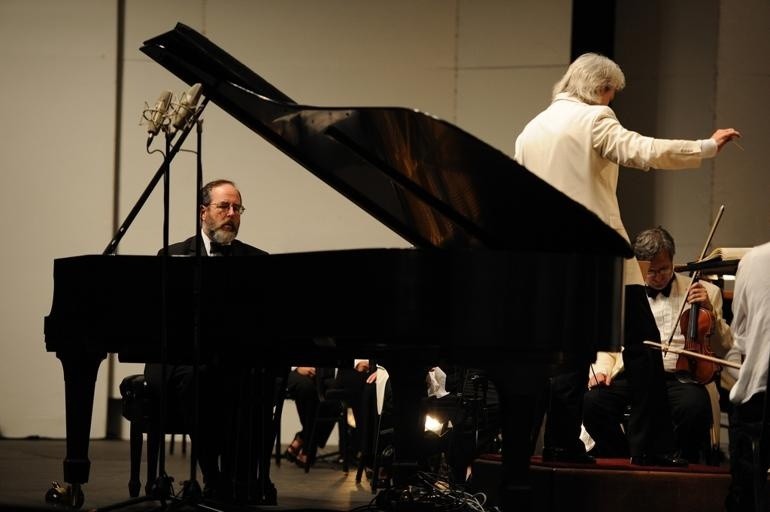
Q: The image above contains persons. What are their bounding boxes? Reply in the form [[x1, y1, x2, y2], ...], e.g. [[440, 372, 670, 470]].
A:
[[514, 49, 741, 472], [582, 223, 735, 464], [282, 355, 382, 472], [724, 242, 770, 510], [142, 177, 271, 498]]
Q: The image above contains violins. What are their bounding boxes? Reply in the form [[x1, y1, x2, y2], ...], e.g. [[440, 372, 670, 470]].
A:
[[674, 255, 722, 383]]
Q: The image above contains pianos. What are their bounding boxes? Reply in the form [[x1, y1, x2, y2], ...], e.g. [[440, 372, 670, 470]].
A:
[[45, 14, 636, 509]]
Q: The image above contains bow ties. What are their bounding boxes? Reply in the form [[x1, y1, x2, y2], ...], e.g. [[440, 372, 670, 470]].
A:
[[646, 281, 673, 300], [208, 241, 233, 256]]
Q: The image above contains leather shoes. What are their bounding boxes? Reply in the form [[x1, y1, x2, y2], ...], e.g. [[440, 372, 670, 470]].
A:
[[543, 448, 596, 465], [628, 450, 689, 466]]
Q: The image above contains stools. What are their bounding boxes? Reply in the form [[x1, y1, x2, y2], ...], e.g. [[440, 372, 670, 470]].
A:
[[120, 372, 248, 498]]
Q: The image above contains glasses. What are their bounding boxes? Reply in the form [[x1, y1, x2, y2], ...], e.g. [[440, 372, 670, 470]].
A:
[[207, 202, 245, 215]]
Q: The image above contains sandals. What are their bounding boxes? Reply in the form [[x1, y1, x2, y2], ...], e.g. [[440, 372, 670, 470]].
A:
[[295, 444, 309, 469], [284, 432, 304, 462]]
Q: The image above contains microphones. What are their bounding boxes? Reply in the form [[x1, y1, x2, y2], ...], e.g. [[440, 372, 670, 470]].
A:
[[147, 91, 172, 133], [172, 83, 205, 129]]
